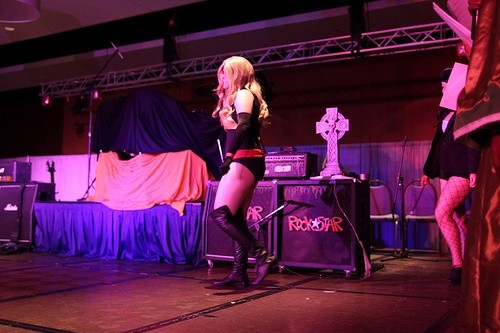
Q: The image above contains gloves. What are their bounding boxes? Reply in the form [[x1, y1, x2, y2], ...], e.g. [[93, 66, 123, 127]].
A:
[[218, 157, 233, 175]]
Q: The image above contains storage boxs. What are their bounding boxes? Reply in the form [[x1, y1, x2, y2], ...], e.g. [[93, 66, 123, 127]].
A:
[[263, 153, 318, 179], [0, 160, 32, 184]]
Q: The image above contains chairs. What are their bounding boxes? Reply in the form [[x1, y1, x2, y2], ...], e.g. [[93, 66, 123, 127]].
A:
[[369, 179, 471, 258]]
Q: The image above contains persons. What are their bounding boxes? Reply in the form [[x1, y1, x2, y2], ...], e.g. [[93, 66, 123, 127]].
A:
[[211, 56, 277, 290], [421, 69, 481, 285]]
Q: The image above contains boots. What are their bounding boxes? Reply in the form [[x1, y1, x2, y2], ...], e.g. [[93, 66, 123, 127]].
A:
[[213, 208, 249, 287], [208, 205, 278, 285]]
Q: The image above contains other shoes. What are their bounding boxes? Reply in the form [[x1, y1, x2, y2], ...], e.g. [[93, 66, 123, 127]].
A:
[[447, 268, 462, 285]]
